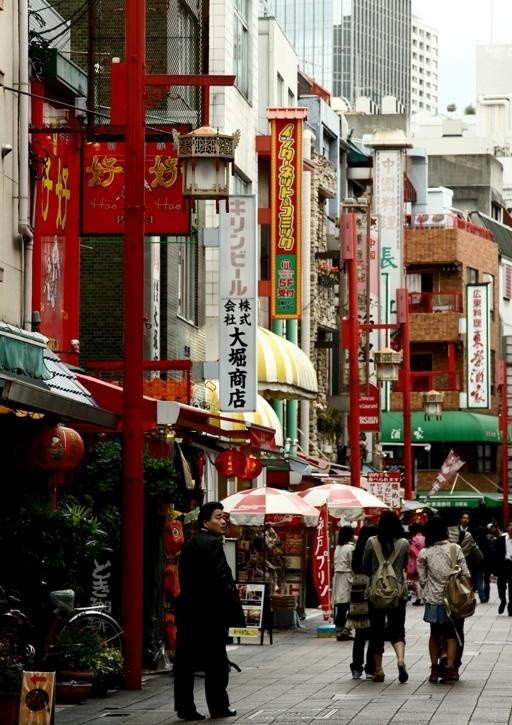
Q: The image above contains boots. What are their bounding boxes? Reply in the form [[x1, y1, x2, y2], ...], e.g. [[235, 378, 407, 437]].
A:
[[428, 665, 454, 684]]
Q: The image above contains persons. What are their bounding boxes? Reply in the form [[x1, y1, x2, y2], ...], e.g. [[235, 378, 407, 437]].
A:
[[332, 511, 512, 683], [174, 500, 246, 720]]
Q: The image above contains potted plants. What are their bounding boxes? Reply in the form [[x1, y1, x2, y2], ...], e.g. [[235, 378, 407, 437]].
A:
[[0, 643, 40, 725], [58, 628, 108, 697]]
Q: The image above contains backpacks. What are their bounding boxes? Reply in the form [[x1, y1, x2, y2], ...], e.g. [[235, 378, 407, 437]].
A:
[[444, 544, 476, 620], [368, 536, 403, 607]]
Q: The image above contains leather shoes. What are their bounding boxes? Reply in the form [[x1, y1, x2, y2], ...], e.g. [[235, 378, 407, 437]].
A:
[[211, 707, 236, 718], [498, 601, 506, 614], [183, 710, 205, 721]]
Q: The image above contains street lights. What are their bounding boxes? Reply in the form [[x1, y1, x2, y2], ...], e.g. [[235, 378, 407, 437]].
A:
[[119, 69, 243, 698], [395, 368, 451, 502], [346, 319, 408, 490]]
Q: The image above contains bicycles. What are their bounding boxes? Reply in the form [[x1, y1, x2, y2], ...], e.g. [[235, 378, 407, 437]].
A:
[[1, 584, 123, 671]]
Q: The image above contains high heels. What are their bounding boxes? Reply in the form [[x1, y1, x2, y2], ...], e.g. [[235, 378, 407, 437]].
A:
[[372, 670, 385, 682], [398, 661, 409, 683]]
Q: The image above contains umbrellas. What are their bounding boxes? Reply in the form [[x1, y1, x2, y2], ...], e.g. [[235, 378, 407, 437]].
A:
[[217, 483, 320, 583], [297, 481, 392, 521]]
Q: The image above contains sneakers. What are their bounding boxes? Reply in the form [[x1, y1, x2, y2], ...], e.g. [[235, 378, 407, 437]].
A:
[[366, 672, 372, 680], [336, 631, 343, 641], [342, 627, 355, 641], [351, 669, 362, 680]]
